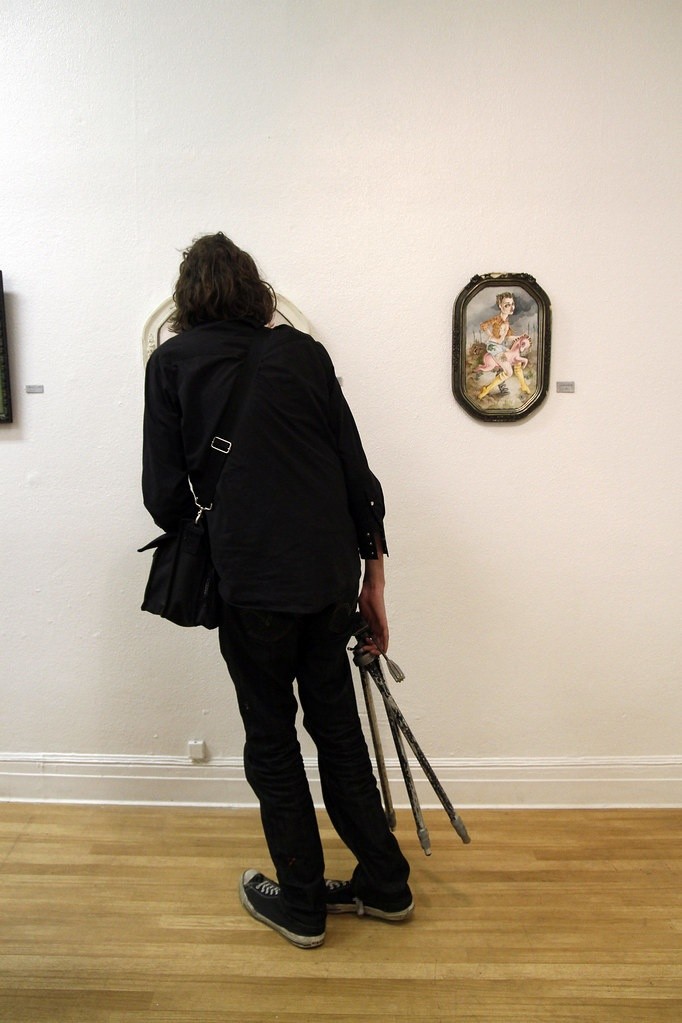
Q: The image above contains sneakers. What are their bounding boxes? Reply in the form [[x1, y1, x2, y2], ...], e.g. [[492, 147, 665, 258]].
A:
[[240, 867, 326, 947], [322, 876, 414, 921]]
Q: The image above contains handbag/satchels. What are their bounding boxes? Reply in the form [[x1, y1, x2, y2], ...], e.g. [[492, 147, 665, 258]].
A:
[[136, 526, 218, 630]]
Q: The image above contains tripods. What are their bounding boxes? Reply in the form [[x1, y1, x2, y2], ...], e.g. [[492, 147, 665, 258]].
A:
[[349, 611, 472, 857]]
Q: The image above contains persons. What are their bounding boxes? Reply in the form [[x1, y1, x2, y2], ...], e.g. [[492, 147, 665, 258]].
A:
[[141, 232, 416, 948]]
